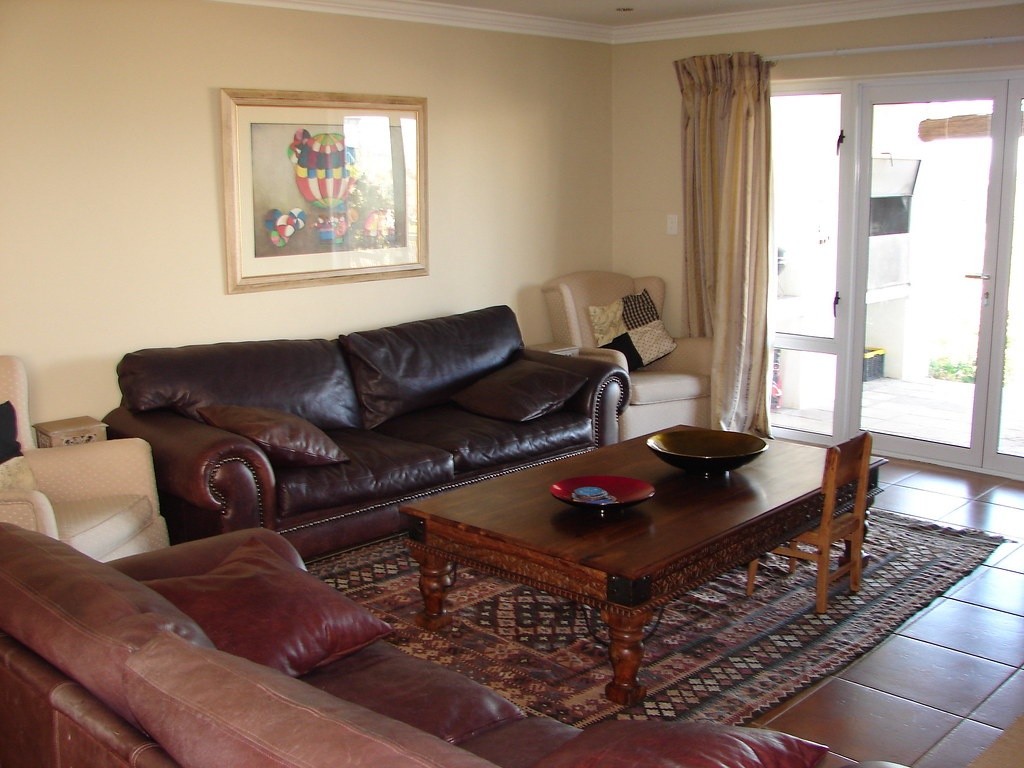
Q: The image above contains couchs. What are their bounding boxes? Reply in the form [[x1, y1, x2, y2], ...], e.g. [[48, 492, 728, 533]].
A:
[[1, 354, 169, 563], [0, 522, 916, 768], [542, 270, 713, 440], [101, 305, 631, 563]]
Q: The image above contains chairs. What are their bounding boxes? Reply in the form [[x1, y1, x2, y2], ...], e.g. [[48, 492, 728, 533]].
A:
[[747, 431, 873, 612]]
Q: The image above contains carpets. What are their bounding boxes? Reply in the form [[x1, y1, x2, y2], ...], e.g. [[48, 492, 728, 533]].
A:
[[308, 506, 1016, 731]]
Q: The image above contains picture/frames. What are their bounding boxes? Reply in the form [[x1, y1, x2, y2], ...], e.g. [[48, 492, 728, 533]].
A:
[[220, 89, 430, 295]]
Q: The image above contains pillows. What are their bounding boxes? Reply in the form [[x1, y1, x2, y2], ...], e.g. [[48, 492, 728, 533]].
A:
[[538, 722, 830, 768], [100, 526, 394, 677], [196, 406, 350, 465], [451, 358, 587, 420], [588, 288, 677, 374]]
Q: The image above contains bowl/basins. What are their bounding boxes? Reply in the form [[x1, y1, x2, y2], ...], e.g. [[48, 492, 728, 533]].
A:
[[646, 428, 769, 479], [548, 475, 655, 512]]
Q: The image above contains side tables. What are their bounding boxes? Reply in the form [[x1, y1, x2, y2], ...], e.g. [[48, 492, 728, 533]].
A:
[[526, 342, 577, 357]]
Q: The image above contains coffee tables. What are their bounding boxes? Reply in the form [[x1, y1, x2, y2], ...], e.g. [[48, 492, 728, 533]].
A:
[[398, 424, 890, 708]]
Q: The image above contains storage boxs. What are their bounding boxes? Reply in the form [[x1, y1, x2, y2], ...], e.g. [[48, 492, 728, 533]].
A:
[[864, 348, 884, 381]]
